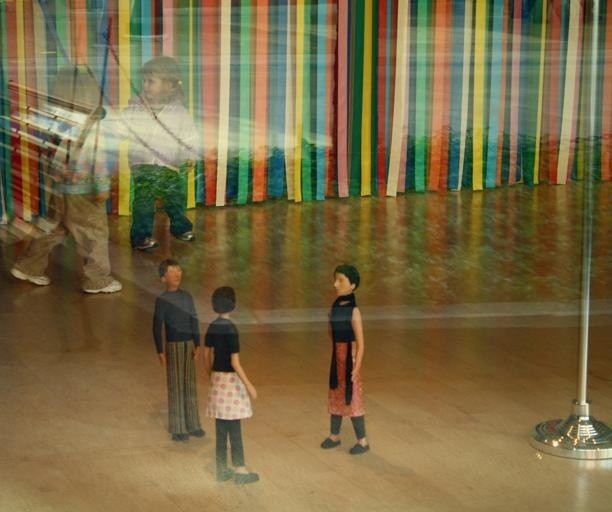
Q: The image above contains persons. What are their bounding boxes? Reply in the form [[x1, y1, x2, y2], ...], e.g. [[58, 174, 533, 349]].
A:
[[153, 260, 205, 442], [204, 286, 263, 485], [321, 263, 371, 454], [113, 59, 199, 248], [10, 63, 122, 294]]
